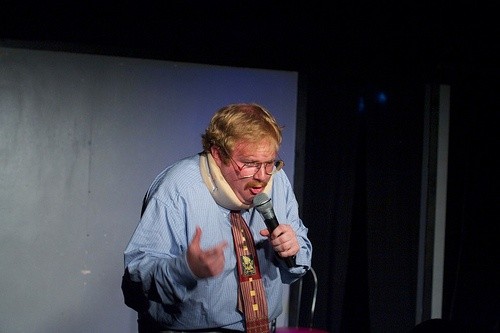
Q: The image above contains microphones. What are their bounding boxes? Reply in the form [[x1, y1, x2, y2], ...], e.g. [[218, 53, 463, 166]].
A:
[[254, 193, 295, 269]]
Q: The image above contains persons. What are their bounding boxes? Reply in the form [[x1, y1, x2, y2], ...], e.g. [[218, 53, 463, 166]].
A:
[[123, 103, 313, 333]]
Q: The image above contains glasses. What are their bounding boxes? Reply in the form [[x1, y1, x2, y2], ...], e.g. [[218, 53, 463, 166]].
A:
[[222, 142, 285, 177]]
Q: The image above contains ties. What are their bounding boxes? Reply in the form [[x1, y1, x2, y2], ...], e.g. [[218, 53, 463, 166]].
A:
[[231, 210, 269, 333]]
